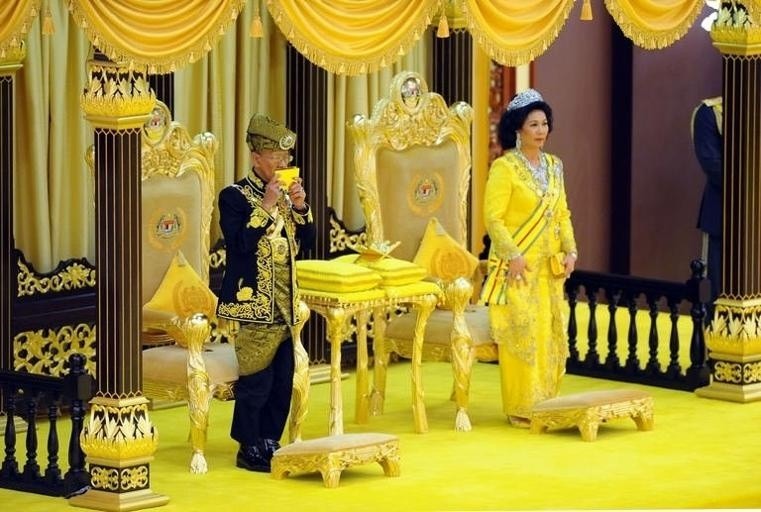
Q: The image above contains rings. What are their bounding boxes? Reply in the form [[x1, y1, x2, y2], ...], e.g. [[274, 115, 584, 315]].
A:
[[516, 273, 522, 280], [301, 186, 304, 193]]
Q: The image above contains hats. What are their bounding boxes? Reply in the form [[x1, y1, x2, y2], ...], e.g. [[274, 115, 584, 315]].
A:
[[246, 113, 297, 153]]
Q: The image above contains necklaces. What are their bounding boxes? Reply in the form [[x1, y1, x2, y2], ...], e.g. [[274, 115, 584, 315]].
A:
[[520, 150, 550, 192]]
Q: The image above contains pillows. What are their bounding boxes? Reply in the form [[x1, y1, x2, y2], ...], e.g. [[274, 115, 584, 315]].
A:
[[411, 217, 481, 310], [139, 249, 219, 348]]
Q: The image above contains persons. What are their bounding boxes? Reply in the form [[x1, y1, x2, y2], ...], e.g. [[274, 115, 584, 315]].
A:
[[692, 93, 723, 372], [480, 90, 578, 430], [214, 112, 313, 472]]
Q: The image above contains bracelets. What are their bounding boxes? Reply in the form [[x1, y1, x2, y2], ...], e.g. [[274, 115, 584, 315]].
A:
[[571, 252, 578, 261], [509, 253, 521, 261]]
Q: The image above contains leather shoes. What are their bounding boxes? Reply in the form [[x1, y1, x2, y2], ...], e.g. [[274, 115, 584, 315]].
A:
[[237, 437, 281, 472], [507, 415, 530, 429]]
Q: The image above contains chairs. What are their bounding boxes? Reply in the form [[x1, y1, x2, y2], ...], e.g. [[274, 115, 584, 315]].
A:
[[343, 71, 573, 433], [84, 100, 312, 476]]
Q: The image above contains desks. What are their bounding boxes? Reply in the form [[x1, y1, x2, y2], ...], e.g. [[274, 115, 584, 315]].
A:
[[293, 281, 438, 436]]
